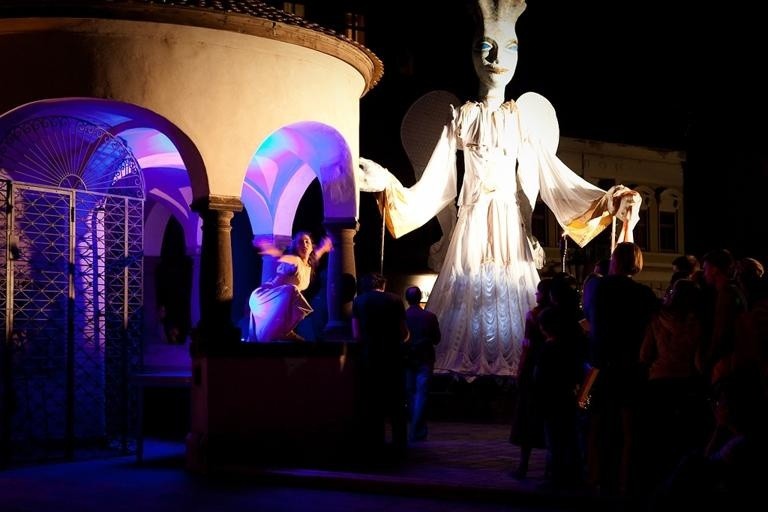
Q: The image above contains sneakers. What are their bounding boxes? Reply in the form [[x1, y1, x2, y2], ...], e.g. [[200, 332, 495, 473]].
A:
[[512, 463, 529, 479], [409, 426, 429, 441]]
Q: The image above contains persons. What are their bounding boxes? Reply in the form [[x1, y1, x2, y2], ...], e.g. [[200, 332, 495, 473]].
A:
[[354, 1, 643, 388], [252, 229, 332, 318], [248, 261, 314, 344], [496, 237, 767, 511], [400, 286, 441, 442], [351, 271, 406, 343]]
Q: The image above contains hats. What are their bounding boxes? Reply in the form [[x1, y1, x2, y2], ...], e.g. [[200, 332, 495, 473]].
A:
[[538, 271, 581, 299], [702, 248, 737, 281]]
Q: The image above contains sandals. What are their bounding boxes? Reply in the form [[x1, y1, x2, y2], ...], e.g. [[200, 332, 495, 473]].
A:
[[280, 329, 305, 342]]
